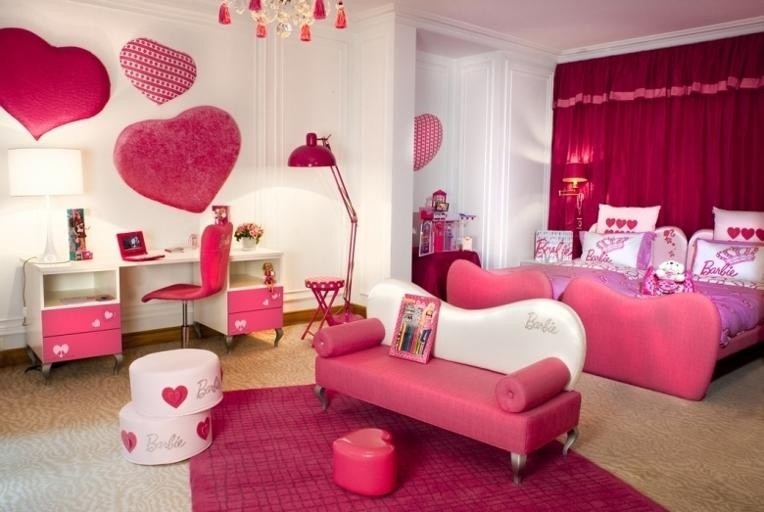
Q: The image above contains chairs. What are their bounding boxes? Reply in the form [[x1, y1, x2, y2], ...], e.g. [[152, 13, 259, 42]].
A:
[[142, 223, 233, 348]]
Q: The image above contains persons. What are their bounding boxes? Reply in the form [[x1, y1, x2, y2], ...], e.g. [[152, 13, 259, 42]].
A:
[[263, 263, 275, 293], [74, 212, 87, 250], [421, 303, 437, 355]]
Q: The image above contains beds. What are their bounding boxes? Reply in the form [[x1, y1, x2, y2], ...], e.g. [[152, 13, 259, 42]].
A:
[[447, 224, 764, 401]]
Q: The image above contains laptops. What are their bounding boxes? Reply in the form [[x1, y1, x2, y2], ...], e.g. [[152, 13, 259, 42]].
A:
[[115, 231, 167, 263]]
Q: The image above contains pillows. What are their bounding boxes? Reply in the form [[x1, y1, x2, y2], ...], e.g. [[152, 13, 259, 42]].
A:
[[578, 231, 656, 271], [691, 238, 764, 282], [596, 203, 662, 233], [712, 205, 764, 243]]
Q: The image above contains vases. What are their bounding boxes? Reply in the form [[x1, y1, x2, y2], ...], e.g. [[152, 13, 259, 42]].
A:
[[240, 238, 256, 250]]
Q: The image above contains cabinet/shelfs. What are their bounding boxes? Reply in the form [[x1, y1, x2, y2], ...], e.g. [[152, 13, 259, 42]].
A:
[[23, 248, 284, 381]]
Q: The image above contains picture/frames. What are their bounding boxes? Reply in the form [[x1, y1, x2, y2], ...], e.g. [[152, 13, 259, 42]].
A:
[[389, 294, 440, 365]]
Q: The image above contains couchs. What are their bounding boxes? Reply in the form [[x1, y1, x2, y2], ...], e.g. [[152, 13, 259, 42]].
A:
[[314, 279, 587, 485]]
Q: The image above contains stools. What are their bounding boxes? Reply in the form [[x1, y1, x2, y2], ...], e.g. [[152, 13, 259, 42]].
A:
[[301, 278, 345, 347], [120, 405, 212, 466], [333, 428, 398, 497], [129, 349, 224, 417]]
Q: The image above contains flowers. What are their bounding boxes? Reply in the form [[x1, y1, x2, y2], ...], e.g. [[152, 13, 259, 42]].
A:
[[235, 223, 263, 244]]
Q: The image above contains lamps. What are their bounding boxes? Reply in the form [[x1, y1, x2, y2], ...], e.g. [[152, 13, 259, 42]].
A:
[[218, 0, 347, 41], [8, 148, 85, 265], [288, 133, 364, 323], [558, 162, 590, 230]]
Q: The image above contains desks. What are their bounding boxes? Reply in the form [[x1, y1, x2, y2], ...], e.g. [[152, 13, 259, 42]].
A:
[[413, 246, 481, 301]]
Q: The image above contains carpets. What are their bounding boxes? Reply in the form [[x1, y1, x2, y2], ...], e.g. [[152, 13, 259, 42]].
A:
[[189, 384, 671, 512]]
